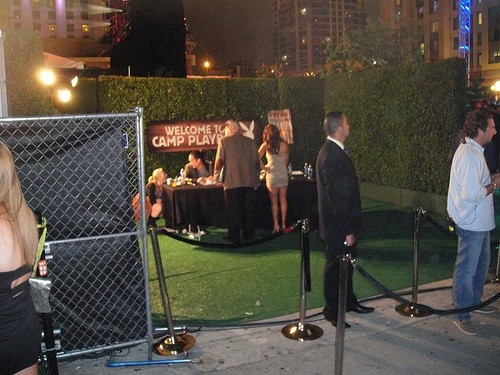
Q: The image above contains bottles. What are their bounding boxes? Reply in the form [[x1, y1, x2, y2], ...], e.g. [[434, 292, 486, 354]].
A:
[[304, 163, 308, 177], [180, 168, 186, 184], [308, 165, 313, 180], [288, 163, 292, 177]]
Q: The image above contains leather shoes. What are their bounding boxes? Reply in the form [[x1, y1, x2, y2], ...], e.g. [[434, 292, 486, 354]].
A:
[[346, 304, 375, 314], [324, 311, 351, 329]]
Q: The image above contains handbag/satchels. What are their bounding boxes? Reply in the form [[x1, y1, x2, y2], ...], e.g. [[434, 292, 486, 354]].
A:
[[132, 181, 154, 223], [218, 164, 225, 182], [29, 277, 52, 313]]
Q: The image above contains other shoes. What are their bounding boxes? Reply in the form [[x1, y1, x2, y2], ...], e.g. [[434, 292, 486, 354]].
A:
[[246, 239, 252, 242], [222, 236, 238, 244], [475, 306, 495, 313], [454, 319, 478, 336]]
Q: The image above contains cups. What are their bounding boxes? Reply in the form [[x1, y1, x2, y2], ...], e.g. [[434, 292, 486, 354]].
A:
[[166, 177, 192, 187]]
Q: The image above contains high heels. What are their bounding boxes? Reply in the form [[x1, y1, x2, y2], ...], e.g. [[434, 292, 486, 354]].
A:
[[280, 225, 285, 231], [272, 226, 280, 234]]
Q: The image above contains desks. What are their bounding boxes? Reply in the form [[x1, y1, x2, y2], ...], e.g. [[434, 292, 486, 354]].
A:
[[163, 173, 316, 232]]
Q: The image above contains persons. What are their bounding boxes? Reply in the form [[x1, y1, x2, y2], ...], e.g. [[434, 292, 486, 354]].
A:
[[146, 168, 169, 229], [182, 151, 210, 185], [447, 109, 500, 334], [314, 112, 374, 328], [0, 140, 41, 375], [258, 123, 289, 235], [212, 120, 260, 242]]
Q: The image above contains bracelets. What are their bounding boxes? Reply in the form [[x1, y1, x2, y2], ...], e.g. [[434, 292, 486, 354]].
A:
[[492, 183, 496, 190]]
